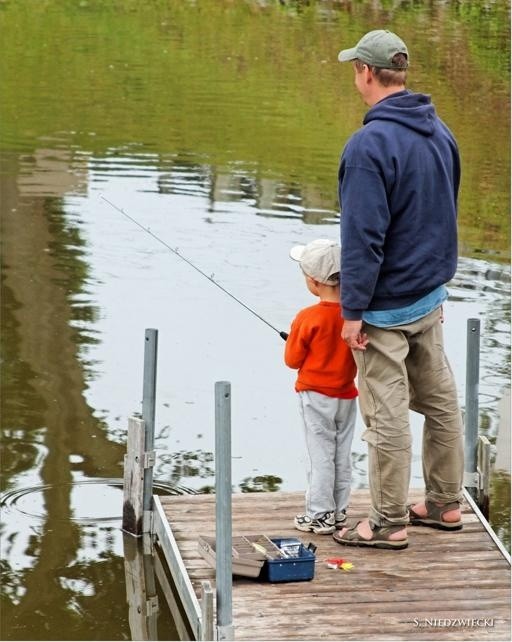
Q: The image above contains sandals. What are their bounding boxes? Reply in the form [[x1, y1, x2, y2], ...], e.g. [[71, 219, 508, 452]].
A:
[[407, 498, 463, 531], [333, 521, 408, 551]]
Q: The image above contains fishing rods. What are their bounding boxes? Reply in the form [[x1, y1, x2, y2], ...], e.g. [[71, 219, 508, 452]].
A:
[[100, 195, 289, 342]]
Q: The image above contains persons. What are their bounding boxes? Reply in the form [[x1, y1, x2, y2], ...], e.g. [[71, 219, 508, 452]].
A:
[[284, 237, 358, 535], [336, 30, 464, 548]]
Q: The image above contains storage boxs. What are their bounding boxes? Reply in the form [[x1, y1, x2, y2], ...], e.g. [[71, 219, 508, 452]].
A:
[[197, 532, 319, 583]]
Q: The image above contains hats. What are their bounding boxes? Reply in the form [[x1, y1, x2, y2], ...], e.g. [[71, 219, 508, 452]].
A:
[[290, 239, 342, 286], [338, 29, 409, 69]]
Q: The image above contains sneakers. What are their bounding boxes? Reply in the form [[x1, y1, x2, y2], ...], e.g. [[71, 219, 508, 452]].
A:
[[334, 510, 348, 529], [294, 512, 336, 535]]
